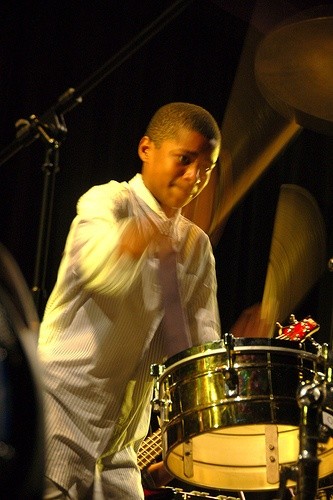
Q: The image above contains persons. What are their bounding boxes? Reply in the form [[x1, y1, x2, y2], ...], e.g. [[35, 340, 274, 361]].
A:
[[36, 101, 223, 500]]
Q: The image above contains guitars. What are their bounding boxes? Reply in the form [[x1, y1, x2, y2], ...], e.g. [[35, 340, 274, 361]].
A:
[[138, 315, 318, 469]]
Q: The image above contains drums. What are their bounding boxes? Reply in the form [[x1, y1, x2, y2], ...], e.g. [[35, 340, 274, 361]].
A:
[[151, 332, 331, 491]]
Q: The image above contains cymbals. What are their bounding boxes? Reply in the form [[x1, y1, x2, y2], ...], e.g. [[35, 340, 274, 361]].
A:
[[255, 17, 333, 121]]
